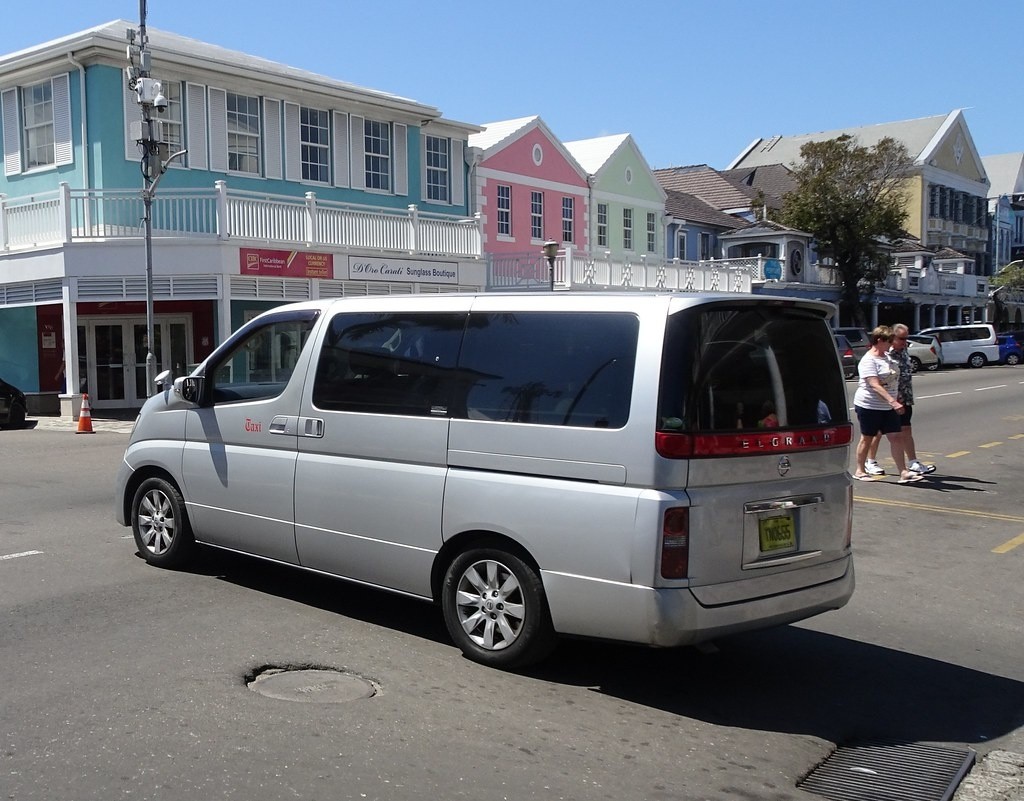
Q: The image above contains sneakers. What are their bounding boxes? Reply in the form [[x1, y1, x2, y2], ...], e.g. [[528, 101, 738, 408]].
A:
[[908, 461, 936, 473], [864, 460, 884, 475]]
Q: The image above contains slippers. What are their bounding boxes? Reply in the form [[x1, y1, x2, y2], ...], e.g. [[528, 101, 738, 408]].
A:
[[851, 472, 877, 482], [896, 470, 924, 484]]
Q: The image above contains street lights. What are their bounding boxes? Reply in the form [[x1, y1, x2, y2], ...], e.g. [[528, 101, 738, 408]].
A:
[[542, 238, 559, 292]]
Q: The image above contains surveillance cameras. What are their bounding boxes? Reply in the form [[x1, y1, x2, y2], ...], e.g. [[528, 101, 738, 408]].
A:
[[153, 95, 168, 113]]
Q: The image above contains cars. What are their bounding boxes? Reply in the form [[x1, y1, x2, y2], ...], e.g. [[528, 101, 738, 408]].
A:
[[906, 341, 938, 375], [995, 334, 1024, 365], [833, 327, 871, 362], [0, 379, 27, 430], [833, 334, 856, 380], [908, 335, 945, 371]]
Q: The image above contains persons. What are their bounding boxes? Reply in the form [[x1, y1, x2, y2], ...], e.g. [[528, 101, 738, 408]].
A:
[[851, 323, 936, 484]]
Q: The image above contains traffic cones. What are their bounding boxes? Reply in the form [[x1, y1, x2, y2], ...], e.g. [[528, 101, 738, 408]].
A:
[[75, 393, 96, 433]]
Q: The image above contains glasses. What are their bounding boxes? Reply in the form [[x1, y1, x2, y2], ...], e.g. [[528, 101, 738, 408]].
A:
[[882, 339, 893, 344], [895, 336, 907, 340]]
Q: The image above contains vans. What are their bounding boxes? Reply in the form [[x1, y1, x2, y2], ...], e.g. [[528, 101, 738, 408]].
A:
[[116, 290, 857, 667], [916, 324, 1001, 369]]
